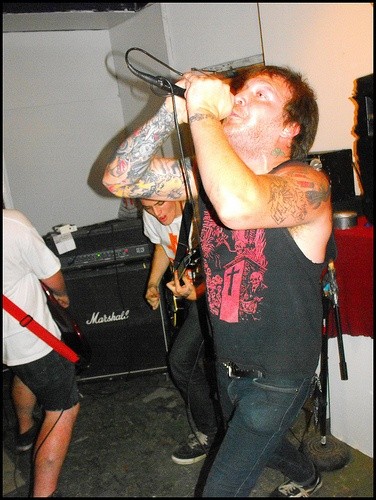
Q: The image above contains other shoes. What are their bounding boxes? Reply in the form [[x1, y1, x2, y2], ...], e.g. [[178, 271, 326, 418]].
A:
[[15, 417, 45, 451]]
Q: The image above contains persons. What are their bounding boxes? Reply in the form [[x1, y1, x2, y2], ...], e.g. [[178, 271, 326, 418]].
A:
[[138, 197, 225, 465], [2, 209, 80, 498], [101, 65, 332, 498]]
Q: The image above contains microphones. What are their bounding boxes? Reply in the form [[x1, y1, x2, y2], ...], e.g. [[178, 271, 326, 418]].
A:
[[157, 77, 228, 125]]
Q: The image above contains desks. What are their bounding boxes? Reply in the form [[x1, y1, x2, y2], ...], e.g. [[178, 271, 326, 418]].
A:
[[328, 217, 373, 459]]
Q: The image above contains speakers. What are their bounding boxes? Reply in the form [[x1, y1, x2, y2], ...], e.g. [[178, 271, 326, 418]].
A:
[[43, 258, 170, 384]]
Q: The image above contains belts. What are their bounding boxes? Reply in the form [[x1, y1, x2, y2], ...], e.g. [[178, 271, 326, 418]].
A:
[[219, 359, 262, 380]]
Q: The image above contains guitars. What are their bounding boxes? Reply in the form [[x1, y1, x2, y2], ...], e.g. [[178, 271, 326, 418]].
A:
[[158, 245, 203, 326], [40, 281, 89, 354]]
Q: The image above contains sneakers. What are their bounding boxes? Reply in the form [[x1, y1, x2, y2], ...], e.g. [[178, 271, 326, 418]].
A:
[[172, 431, 212, 466], [269, 469, 323, 498]]
